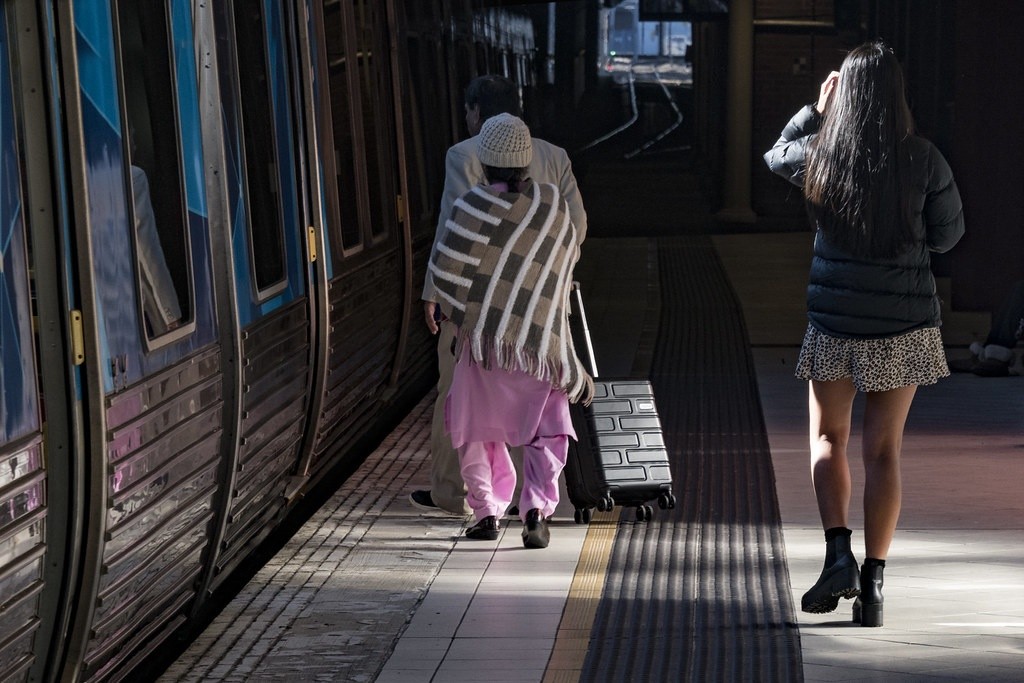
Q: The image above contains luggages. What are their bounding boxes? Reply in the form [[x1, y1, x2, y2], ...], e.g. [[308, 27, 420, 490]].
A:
[[563, 269, 677, 524]]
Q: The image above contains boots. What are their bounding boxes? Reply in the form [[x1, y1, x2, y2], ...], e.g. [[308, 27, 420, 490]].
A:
[[800, 529, 885, 628]]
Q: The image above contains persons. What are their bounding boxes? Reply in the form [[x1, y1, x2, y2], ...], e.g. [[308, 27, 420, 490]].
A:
[[433, 109, 593, 548], [942, 284, 1023, 376], [763, 33, 965, 626], [410, 75, 587, 517]]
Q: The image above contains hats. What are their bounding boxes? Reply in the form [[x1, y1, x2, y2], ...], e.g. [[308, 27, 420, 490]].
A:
[[475, 112, 533, 167]]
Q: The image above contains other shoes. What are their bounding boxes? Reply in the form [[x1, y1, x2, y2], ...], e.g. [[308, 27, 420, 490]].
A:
[[408, 489, 462, 517], [521, 507, 549, 547], [466, 515, 499, 541]]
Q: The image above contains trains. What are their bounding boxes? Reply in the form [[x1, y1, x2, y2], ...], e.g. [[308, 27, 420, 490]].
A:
[[0, 0, 588, 683]]
